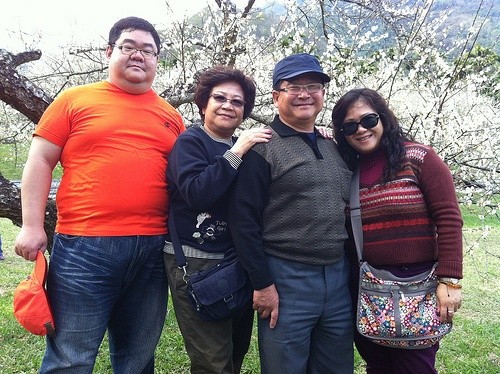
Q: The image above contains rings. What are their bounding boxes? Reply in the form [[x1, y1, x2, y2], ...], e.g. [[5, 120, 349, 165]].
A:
[[447, 311, 454, 313]]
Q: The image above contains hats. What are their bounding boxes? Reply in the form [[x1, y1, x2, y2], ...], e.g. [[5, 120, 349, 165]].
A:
[[14, 250, 56, 337], [273, 53, 330, 89]]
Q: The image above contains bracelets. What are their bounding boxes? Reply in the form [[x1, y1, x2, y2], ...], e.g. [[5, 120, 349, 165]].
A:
[[440, 281, 462, 296], [440, 277, 459, 284]]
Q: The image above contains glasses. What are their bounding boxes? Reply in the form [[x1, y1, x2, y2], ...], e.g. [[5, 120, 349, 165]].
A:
[[208, 94, 247, 107], [340, 113, 379, 135], [276, 83, 325, 94], [113, 44, 157, 59]]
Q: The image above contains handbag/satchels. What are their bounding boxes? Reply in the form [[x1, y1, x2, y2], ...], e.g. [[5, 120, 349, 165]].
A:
[[356, 262, 452, 349], [187, 252, 253, 322]]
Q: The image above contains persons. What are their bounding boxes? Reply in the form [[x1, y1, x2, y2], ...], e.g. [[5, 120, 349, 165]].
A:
[[332, 89, 463, 374], [162, 65, 273, 374], [15, 17, 186, 374], [230, 54, 356, 374]]
